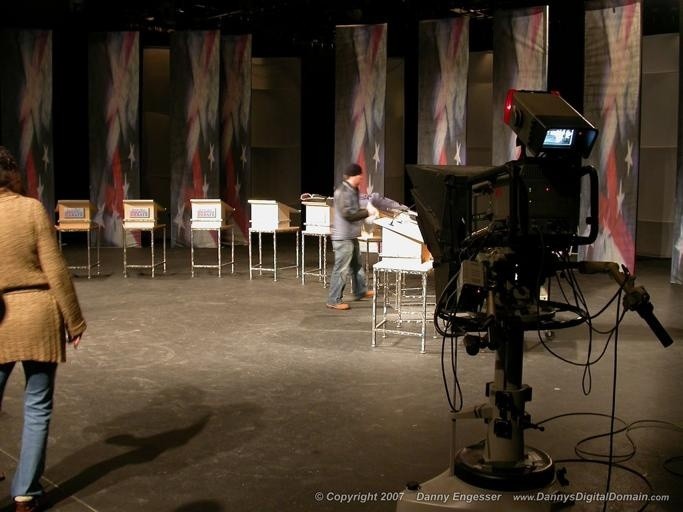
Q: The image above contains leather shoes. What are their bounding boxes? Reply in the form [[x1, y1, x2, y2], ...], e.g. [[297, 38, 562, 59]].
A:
[[362, 289, 374, 298], [326, 301, 350, 310]]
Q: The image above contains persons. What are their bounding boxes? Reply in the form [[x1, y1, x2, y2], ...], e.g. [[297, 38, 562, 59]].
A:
[[324, 163, 378, 310], [0, 148, 87, 511]]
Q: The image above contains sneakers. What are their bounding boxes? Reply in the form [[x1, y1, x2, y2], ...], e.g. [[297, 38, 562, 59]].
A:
[[14, 497, 38, 512]]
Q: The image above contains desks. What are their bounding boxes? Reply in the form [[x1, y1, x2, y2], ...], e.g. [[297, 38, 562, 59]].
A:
[[369, 253, 440, 355], [57, 224, 383, 295]]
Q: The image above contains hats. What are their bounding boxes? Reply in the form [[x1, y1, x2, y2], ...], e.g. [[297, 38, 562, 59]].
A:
[[342, 164, 362, 176]]
